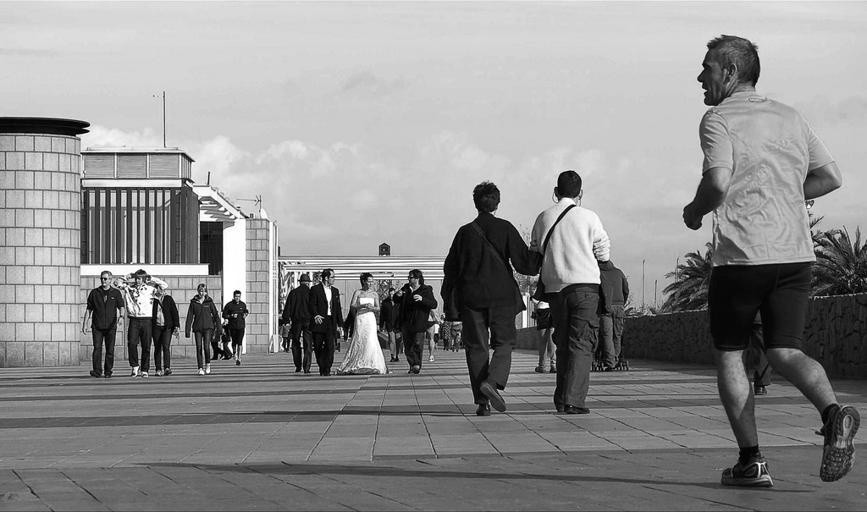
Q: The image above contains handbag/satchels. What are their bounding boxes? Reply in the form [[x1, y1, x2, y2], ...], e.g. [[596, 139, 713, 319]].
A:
[[534, 204, 577, 302], [535, 308, 556, 330]]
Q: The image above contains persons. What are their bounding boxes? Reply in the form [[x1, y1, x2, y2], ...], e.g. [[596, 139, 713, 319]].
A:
[[392, 269, 437, 375], [115, 270, 167, 377], [81, 270, 123, 379], [308, 267, 343, 378], [598, 259, 630, 367], [152, 285, 180, 377], [282, 276, 313, 375], [530, 269, 558, 373], [224, 290, 248, 366], [446, 182, 544, 416], [531, 171, 609, 415], [427, 308, 440, 363], [379, 287, 403, 363], [336, 272, 388, 375], [281, 321, 291, 352], [221, 319, 233, 360], [211, 310, 224, 363], [682, 35, 860, 487], [185, 283, 219, 375], [450, 321, 462, 353], [438, 313, 450, 351], [742, 311, 772, 394]]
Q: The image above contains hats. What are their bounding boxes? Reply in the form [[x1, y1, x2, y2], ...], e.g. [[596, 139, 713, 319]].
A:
[[298, 274, 312, 282]]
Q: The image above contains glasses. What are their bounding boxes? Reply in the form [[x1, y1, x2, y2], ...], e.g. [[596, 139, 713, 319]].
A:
[[100, 277, 108, 280], [330, 275, 335, 278], [409, 276, 415, 279]]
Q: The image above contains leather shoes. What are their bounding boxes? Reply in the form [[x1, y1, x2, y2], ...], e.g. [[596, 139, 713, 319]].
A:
[[755, 386, 768, 396]]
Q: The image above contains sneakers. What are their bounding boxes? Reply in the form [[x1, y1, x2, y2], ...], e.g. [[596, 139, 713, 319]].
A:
[[564, 405, 590, 414], [105, 372, 111, 378], [813, 405, 860, 482], [90, 371, 101, 377], [481, 380, 507, 412], [198, 370, 204, 375], [141, 372, 148, 378], [164, 368, 172, 375], [206, 365, 210, 374], [476, 406, 491, 416], [155, 369, 162, 376], [721, 456, 773, 488], [131, 366, 140, 378]]
